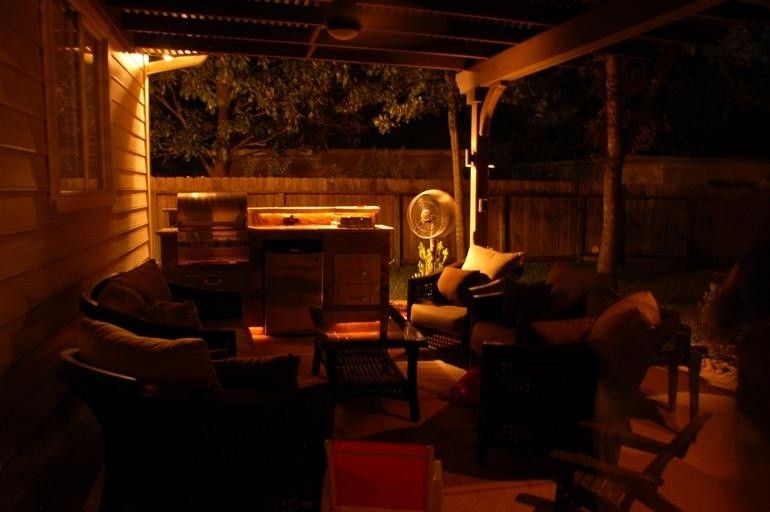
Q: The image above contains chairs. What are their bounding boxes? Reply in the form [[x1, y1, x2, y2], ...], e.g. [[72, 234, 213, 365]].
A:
[[80, 259, 246, 357], [649, 264, 741, 414], [462, 260, 613, 365], [408, 243, 527, 369], [476, 292, 662, 455], [547, 409, 712, 511], [56, 316, 334, 511]]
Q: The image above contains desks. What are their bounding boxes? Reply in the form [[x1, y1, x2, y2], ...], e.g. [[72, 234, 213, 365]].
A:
[[308, 305, 429, 419]]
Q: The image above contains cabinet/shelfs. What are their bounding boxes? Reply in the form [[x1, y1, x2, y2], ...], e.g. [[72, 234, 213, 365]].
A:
[[156, 192, 396, 335]]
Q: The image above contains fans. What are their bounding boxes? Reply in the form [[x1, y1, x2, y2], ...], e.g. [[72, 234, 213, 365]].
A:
[[407, 187, 458, 275]]
